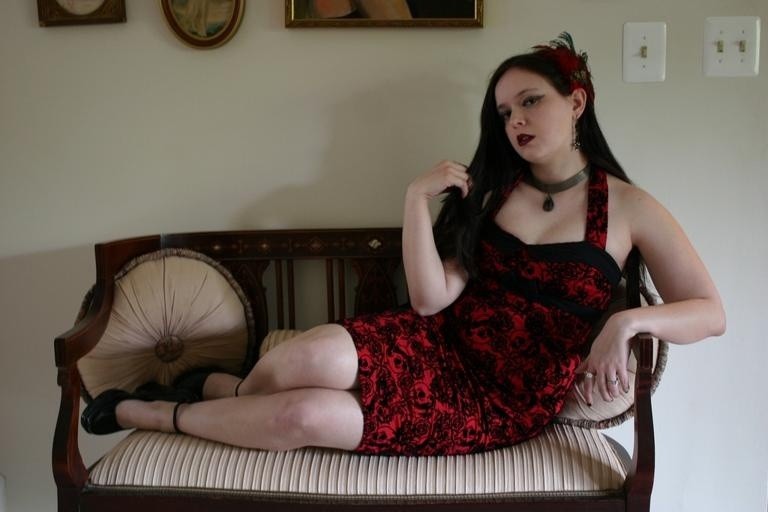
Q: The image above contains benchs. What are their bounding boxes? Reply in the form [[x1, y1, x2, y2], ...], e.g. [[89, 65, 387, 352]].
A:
[[51, 228, 654, 511]]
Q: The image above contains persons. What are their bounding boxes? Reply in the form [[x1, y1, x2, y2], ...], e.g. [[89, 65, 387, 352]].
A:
[[81, 32, 726, 455]]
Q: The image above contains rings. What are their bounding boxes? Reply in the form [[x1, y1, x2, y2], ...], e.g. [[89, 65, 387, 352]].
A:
[[607, 380, 618, 386], [582, 370, 594, 378]]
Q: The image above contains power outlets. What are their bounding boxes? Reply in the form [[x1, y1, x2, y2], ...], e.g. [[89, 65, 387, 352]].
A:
[[623, 17, 760, 84]]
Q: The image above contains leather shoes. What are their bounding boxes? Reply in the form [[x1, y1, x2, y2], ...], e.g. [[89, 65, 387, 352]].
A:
[[80, 381, 199, 434], [172, 366, 244, 401]]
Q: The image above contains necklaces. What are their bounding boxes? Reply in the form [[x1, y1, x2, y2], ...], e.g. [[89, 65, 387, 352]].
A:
[[521, 162, 591, 212]]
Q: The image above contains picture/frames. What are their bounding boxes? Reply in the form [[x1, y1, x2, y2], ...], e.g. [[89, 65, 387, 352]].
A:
[[37, 0, 128, 27], [285, 0, 485, 28], [160, 0, 247, 51]]
